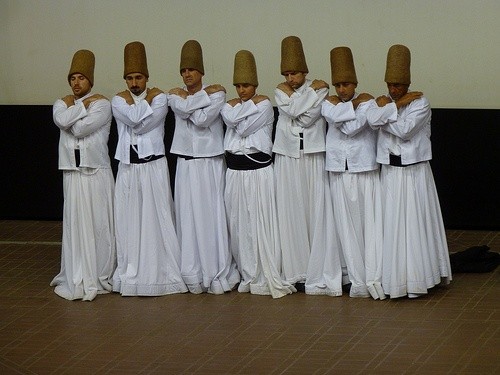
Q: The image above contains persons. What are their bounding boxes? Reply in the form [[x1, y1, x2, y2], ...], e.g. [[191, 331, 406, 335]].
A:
[[272, 36, 330, 285], [166, 39, 240, 294], [110, 41, 188, 296], [220, 50, 297, 299], [50, 49, 114, 301], [367, 44, 452, 299], [305, 47, 387, 300]]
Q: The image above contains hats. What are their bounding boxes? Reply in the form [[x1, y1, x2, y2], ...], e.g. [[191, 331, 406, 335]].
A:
[[180, 40, 204, 76], [232, 50, 258, 87], [68, 49, 95, 88], [124, 41, 149, 79], [330, 47, 358, 85], [384, 45, 411, 84], [281, 36, 309, 75]]
